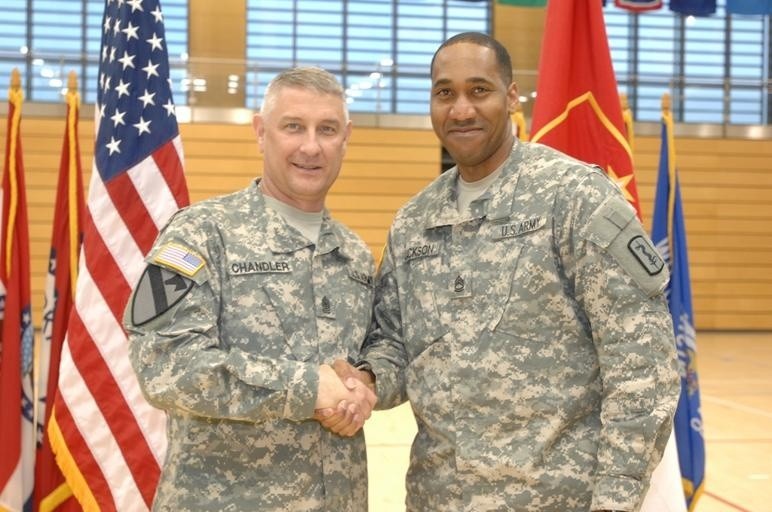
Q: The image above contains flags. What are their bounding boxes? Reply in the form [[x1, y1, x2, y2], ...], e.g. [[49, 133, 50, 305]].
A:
[[529, 1, 645, 230], [35, 1, 194, 512], [651, 114, 709, 511], [0, 90, 37, 512], [36, 93, 87, 448]]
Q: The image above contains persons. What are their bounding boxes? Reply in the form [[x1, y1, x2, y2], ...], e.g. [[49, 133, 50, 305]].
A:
[[123, 65, 381, 512], [330, 31, 685, 512]]
